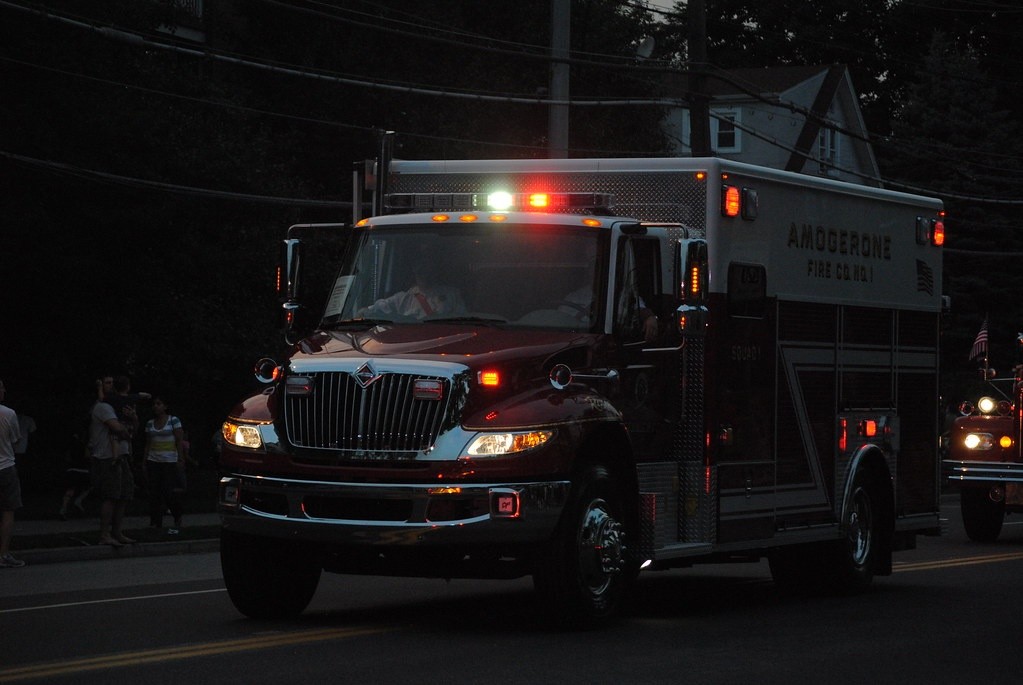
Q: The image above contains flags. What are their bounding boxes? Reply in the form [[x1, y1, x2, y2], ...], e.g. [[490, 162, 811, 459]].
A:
[[968, 317, 988, 359]]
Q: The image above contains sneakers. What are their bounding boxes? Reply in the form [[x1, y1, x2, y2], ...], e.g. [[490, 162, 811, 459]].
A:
[[0, 553, 25, 567]]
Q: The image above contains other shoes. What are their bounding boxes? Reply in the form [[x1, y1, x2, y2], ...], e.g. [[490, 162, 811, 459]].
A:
[[97, 532, 125, 547], [109, 455, 127, 470], [111, 532, 137, 543]]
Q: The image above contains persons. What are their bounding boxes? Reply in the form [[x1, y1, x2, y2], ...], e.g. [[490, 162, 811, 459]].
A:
[[356, 255, 470, 321], [556, 241, 659, 344], [0, 373, 220, 567]]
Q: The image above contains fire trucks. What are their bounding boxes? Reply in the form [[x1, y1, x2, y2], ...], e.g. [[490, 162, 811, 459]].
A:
[[215, 128, 947, 638], [938, 362, 1023, 544]]
